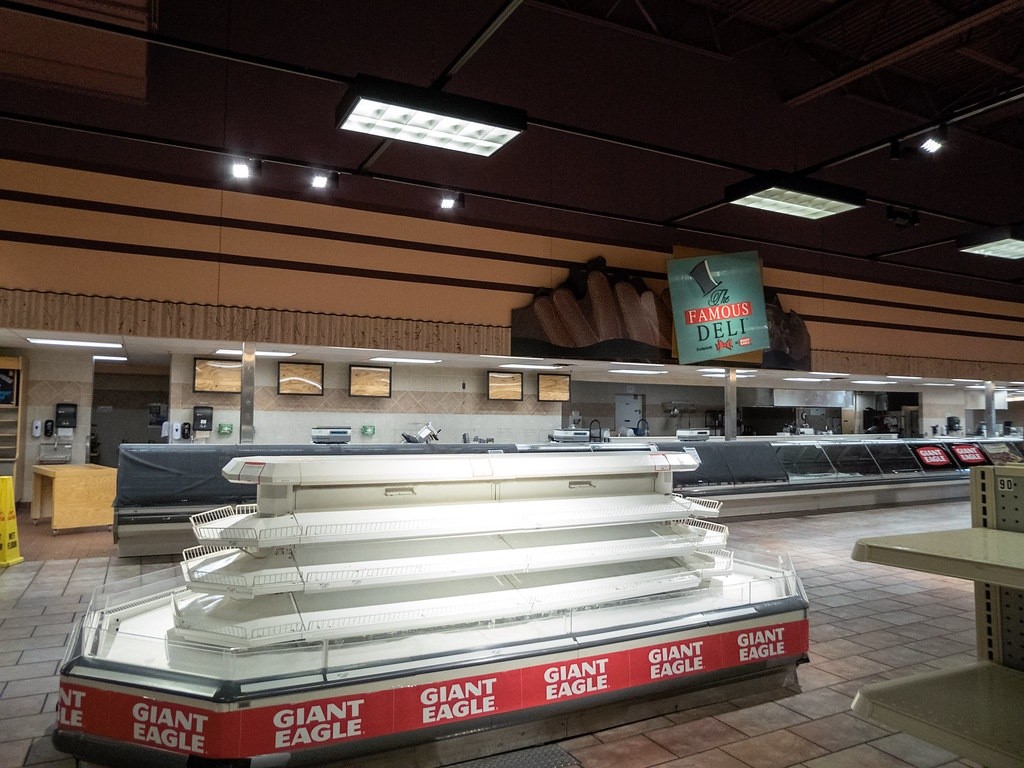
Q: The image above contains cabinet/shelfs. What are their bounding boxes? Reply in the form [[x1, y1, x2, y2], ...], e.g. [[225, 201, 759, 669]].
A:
[[0, 355, 30, 510], [852, 525, 1024, 768]]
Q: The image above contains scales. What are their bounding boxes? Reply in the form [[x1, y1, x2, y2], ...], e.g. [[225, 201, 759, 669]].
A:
[[675, 428, 710, 442], [310, 425, 351, 444], [549, 428, 590, 442]]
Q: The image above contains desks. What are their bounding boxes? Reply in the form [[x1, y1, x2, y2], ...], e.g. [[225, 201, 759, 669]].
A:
[[30, 463, 118, 537]]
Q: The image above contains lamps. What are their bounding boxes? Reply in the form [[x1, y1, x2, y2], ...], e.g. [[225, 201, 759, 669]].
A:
[[916, 122, 949, 155], [335, 70, 529, 158], [233, 159, 263, 178], [313, 171, 340, 189], [888, 141, 902, 161], [725, 169, 868, 220], [440, 193, 466, 209], [884, 205, 922, 227], [956, 225, 1024, 260]]
